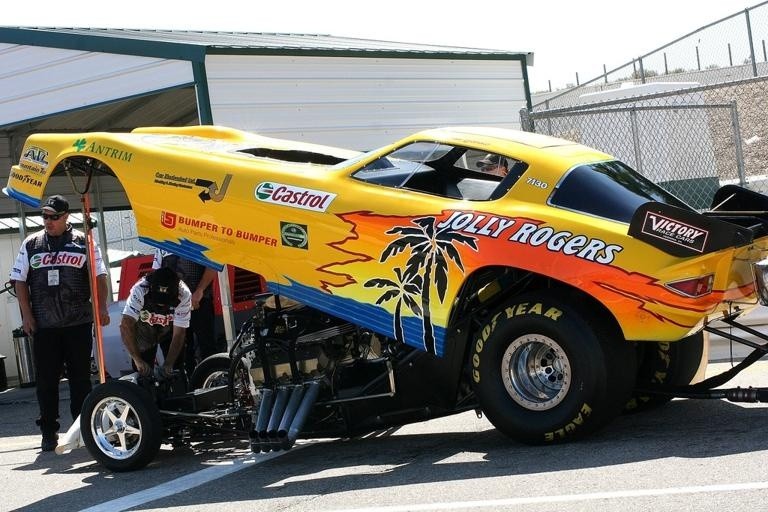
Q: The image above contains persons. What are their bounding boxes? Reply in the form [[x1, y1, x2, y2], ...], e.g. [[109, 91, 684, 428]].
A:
[[476, 153, 509, 177], [151, 247, 218, 373], [119, 267, 193, 378], [9, 195, 110, 452]]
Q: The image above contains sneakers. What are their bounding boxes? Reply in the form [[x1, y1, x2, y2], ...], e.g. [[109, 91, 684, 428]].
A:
[[41, 430, 59, 452]]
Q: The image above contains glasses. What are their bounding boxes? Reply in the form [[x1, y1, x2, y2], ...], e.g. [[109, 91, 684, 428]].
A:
[[41, 211, 64, 221]]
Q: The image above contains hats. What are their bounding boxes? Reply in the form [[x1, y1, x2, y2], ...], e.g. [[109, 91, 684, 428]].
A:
[[476, 152, 508, 171], [40, 194, 70, 214], [146, 267, 183, 306]]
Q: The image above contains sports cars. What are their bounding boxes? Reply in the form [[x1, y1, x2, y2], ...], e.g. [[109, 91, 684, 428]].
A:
[[4, 124, 768, 472]]
